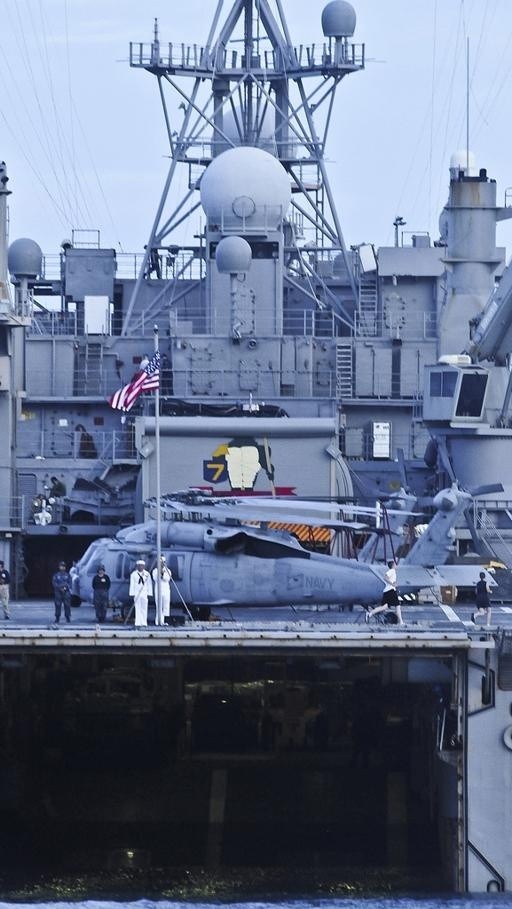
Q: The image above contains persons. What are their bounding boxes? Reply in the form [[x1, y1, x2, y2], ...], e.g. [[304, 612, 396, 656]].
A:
[[33, 493, 52, 527], [162, 353, 173, 396], [365, 561, 405, 625], [49, 477, 66, 498], [129, 560, 153, 627], [92, 566, 111, 622], [0, 560, 12, 620], [139, 353, 149, 370], [52, 560, 71, 624], [143, 248, 162, 280], [152, 556, 172, 625], [471, 572, 493, 626]]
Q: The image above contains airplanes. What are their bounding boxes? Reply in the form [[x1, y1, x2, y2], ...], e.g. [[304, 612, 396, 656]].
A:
[[71, 484, 504, 618]]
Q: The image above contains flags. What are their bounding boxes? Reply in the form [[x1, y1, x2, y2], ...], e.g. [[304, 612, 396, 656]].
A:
[[108, 350, 161, 412]]
